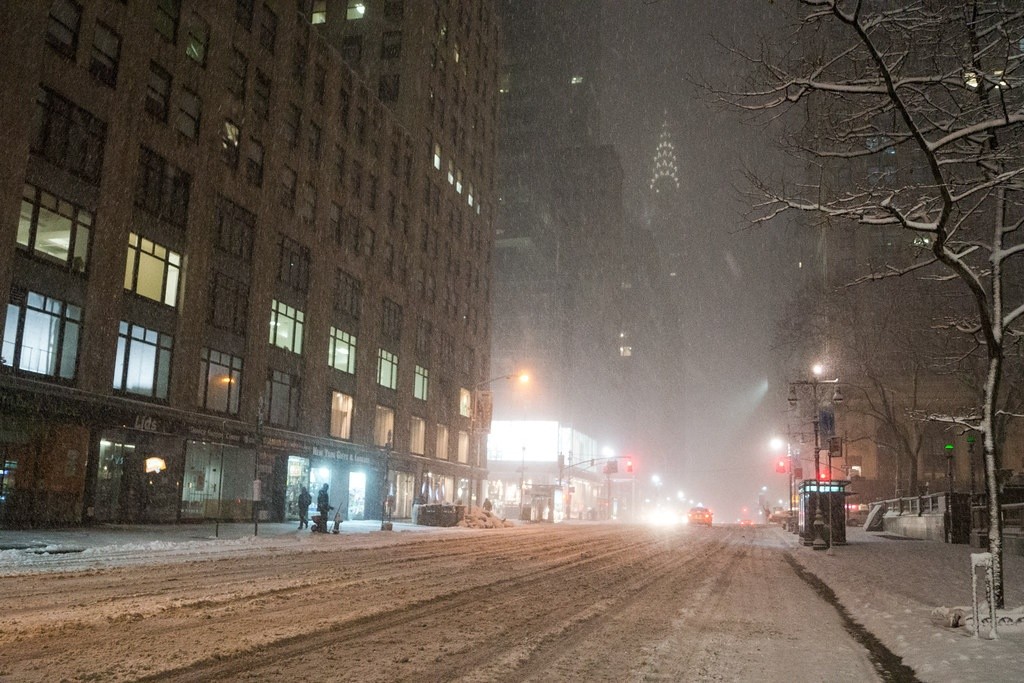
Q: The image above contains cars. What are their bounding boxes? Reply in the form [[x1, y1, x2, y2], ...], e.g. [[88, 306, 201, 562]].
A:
[[688, 506, 714, 526]]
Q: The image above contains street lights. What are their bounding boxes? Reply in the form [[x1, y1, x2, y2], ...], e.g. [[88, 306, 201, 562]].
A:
[[788, 375, 846, 549], [966, 435, 977, 529], [944, 443, 958, 544], [467, 369, 530, 514]]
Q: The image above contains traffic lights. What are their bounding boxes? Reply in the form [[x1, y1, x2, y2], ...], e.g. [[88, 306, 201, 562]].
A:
[[627, 461, 633, 472], [820, 474, 826, 479], [776, 460, 786, 473]]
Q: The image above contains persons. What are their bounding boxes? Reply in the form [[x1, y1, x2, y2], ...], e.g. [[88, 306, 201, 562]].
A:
[[483, 498, 492, 512], [297, 487, 312, 529], [317, 484, 334, 534]]
